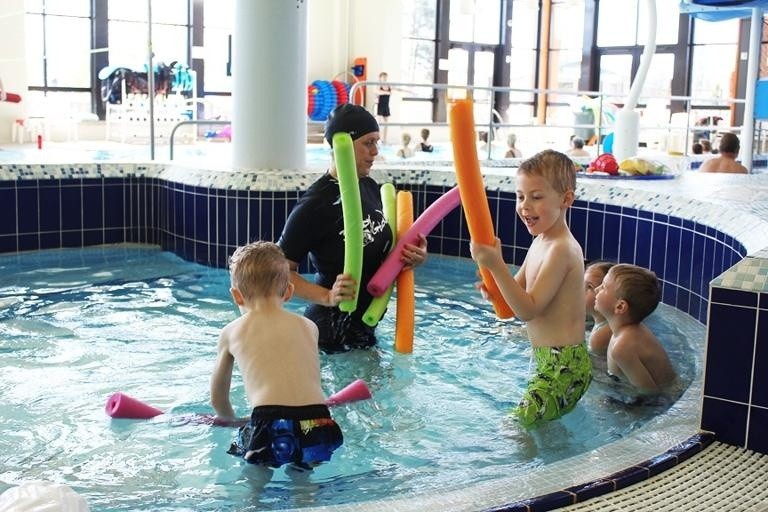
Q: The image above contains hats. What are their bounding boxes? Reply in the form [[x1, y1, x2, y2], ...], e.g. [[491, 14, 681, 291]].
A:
[[324, 104, 379, 148]]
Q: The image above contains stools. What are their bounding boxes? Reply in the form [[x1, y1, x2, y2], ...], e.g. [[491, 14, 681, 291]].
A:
[[12, 116, 50, 144]]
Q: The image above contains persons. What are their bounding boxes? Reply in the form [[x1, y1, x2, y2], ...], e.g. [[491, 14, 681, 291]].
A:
[[505, 133, 522, 158], [210, 240, 343, 490], [692, 134, 748, 173], [397, 134, 417, 161], [569, 136, 590, 157], [594, 263, 677, 397], [276, 103, 428, 353], [419, 129, 434, 153], [375, 72, 392, 144], [586, 263, 613, 359], [469, 150, 593, 460]]
